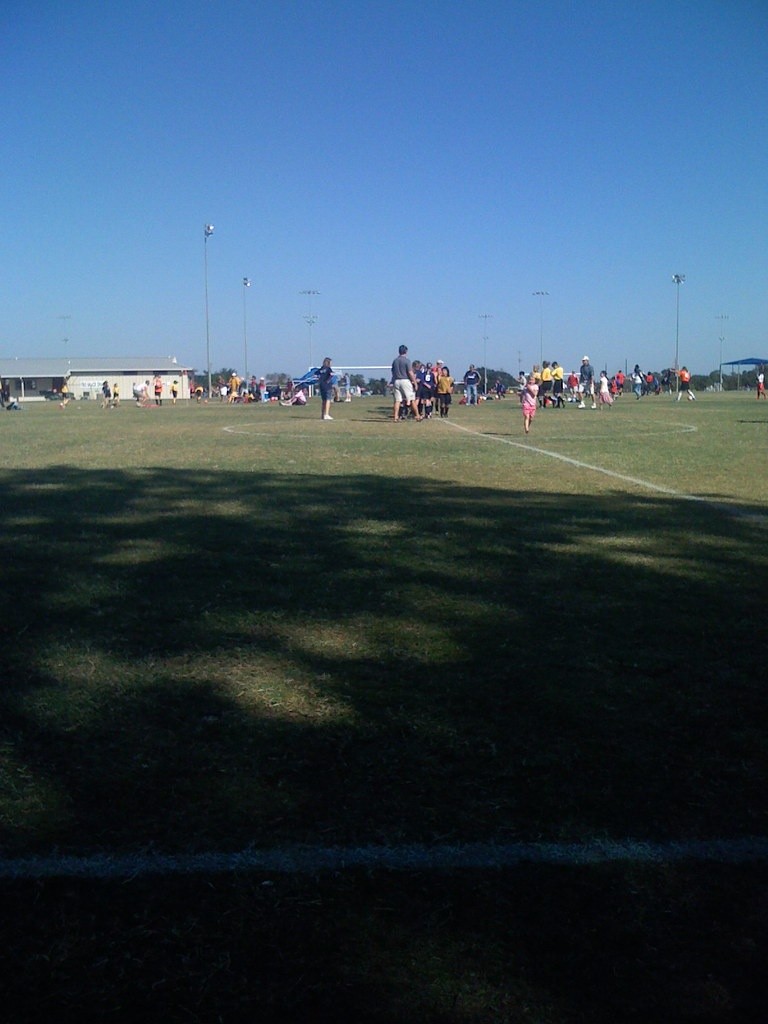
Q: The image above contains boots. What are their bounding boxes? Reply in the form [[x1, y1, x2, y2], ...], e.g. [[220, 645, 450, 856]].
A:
[[444, 406, 449, 417], [441, 407, 445, 418]]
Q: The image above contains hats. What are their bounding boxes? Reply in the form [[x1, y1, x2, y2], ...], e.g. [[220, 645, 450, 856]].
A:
[[232, 373, 236, 377], [437, 360, 444, 363], [582, 355, 589, 360]]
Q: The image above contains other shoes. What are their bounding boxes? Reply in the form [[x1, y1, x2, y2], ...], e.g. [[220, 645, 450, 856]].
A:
[[563, 399, 566, 408], [554, 405, 560, 407], [553, 400, 556, 407], [416, 414, 424, 422], [324, 415, 333, 420], [591, 403, 597, 408], [394, 417, 405, 422], [578, 401, 585, 408], [345, 398, 351, 402]]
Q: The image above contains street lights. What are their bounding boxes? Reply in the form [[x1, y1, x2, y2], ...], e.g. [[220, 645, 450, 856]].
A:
[[671, 274, 687, 392], [300, 290, 320, 370], [715, 314, 730, 391], [243, 277, 251, 392], [479, 313, 494, 394], [203, 224, 215, 398], [531, 291, 550, 365]]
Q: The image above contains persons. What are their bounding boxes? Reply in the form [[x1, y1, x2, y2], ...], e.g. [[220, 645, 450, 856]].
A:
[[392, 345, 455, 423], [517, 356, 626, 410], [496, 379, 505, 399], [520, 375, 539, 434], [344, 373, 351, 402], [217, 373, 266, 404], [632, 364, 696, 402], [319, 358, 333, 420], [7, 401, 25, 410], [0, 379, 9, 407], [463, 364, 482, 406], [756, 370, 766, 399], [102, 376, 203, 409], [279, 378, 306, 406]]
